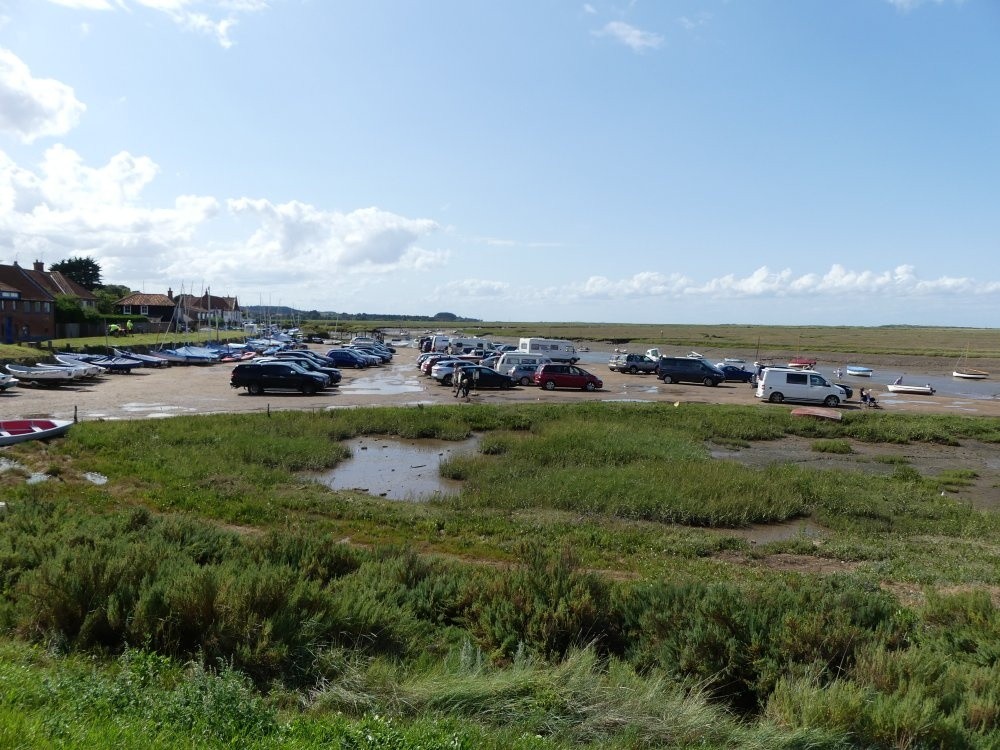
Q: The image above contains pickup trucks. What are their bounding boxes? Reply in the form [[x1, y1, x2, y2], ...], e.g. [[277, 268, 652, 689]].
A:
[[230, 350, 342, 396]]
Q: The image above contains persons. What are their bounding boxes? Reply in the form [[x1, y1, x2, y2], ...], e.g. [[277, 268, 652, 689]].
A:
[[804, 363, 814, 370], [859, 386, 880, 409], [450, 362, 469, 398], [374, 333, 384, 343], [227, 318, 243, 330], [740, 365, 745, 372], [661, 330, 663, 337], [177, 323, 194, 333], [107, 320, 144, 338], [837, 368, 843, 382], [14, 321, 20, 336], [894, 375, 903, 385], [432, 347, 436, 353], [292, 338, 297, 344]]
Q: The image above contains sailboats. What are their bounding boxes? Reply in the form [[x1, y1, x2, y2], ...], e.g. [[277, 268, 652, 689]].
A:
[[952, 339, 991, 379], [149, 277, 323, 365], [752, 336, 776, 389], [788, 336, 816, 370]]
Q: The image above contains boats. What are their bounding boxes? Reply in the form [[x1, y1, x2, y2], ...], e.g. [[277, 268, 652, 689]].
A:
[[398, 324, 463, 336], [714, 358, 746, 370], [645, 348, 703, 362], [578, 346, 590, 352], [847, 365, 875, 378], [0, 346, 169, 390], [886, 377, 937, 395], [0, 416, 74, 448]]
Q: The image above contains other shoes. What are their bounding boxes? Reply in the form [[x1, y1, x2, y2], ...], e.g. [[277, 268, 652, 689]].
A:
[[452, 391, 456, 392], [454, 396, 457, 397], [462, 396, 464, 398], [466, 400, 470, 403]]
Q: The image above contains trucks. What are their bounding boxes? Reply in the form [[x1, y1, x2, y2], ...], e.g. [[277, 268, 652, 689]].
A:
[[418, 336, 498, 351], [519, 338, 581, 365]]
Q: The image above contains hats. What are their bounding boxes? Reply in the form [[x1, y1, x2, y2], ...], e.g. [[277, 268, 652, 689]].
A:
[[458, 368, 462, 371], [463, 379, 468, 384]]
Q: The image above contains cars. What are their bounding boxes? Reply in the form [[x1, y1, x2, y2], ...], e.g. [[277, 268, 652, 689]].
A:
[[327, 336, 396, 368], [813, 378, 853, 398], [416, 344, 539, 391], [720, 366, 754, 383]]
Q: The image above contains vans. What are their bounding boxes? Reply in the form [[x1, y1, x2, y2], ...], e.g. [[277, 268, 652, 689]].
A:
[[756, 367, 847, 408], [656, 356, 725, 386], [497, 353, 552, 374], [532, 363, 603, 391]]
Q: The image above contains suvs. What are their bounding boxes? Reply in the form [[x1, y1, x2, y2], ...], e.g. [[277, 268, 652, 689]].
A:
[[608, 353, 660, 375]]
